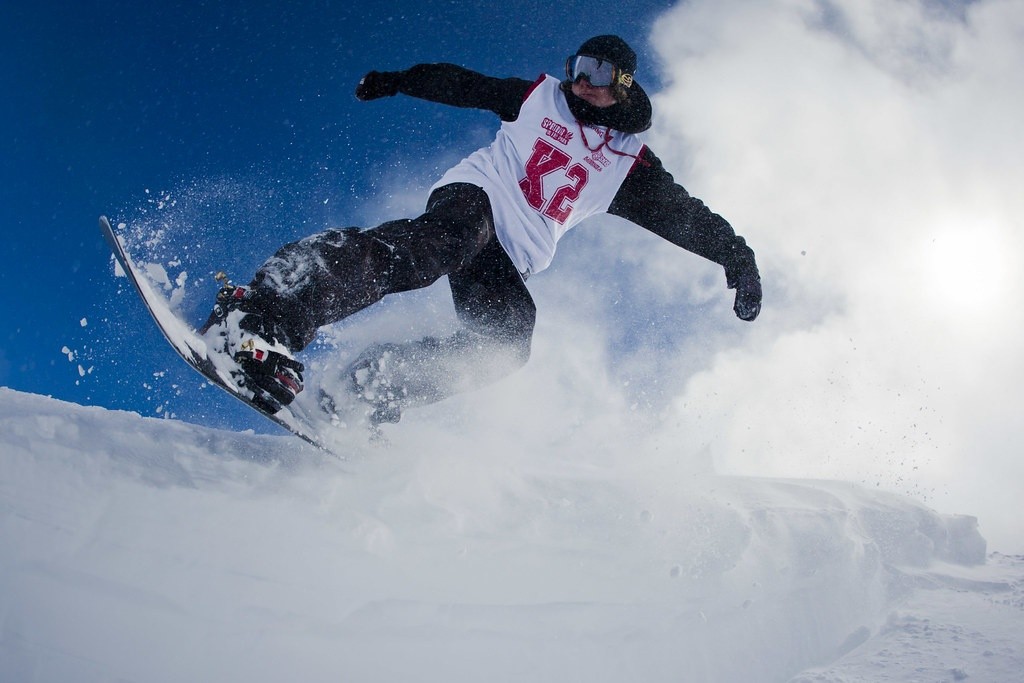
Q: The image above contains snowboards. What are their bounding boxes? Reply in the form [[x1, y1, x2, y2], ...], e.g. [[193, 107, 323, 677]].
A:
[[99, 214, 363, 467]]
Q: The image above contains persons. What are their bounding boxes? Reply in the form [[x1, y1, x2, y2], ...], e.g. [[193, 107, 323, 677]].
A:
[[195, 35, 762, 446]]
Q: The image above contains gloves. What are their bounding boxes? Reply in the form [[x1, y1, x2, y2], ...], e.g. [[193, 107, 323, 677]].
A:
[[355, 70, 399, 101], [724, 261, 763, 322]]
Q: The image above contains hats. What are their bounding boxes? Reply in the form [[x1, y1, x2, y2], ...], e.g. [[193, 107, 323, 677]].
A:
[[576, 35, 637, 76]]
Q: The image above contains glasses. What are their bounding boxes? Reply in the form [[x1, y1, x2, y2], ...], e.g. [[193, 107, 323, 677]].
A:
[[566, 55, 633, 89]]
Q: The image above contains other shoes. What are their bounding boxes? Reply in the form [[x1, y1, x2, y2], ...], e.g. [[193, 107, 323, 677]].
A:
[[223, 286, 303, 406]]
